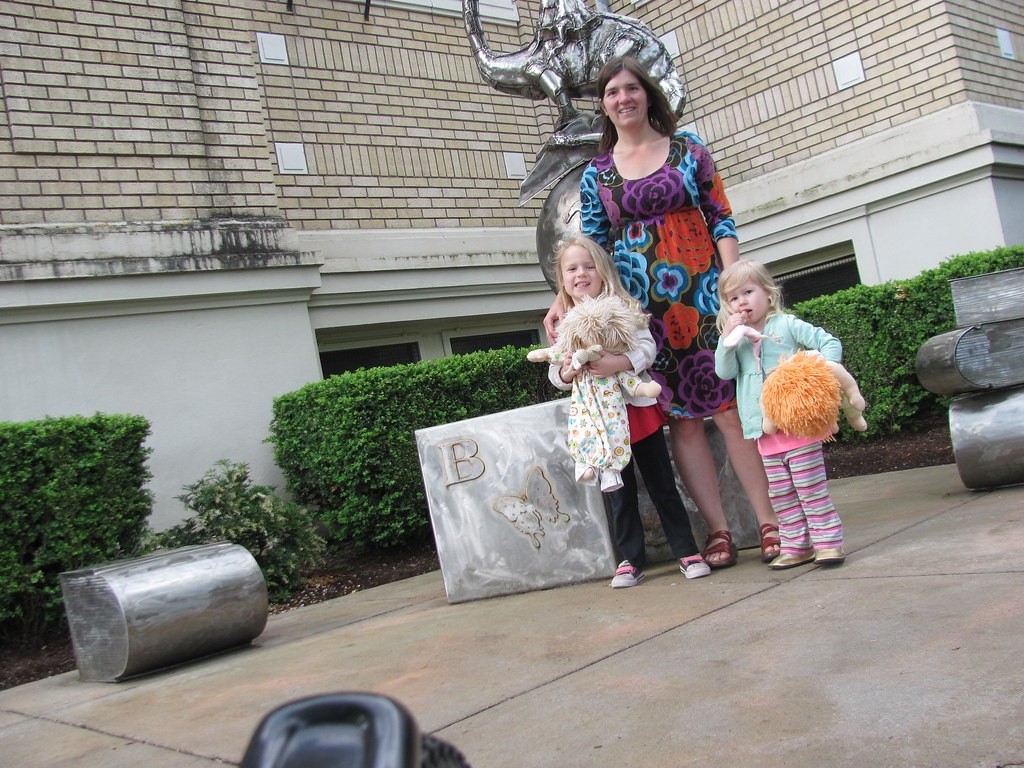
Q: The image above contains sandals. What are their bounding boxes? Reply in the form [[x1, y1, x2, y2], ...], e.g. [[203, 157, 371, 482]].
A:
[[759, 523, 782, 562], [703, 529, 739, 568]]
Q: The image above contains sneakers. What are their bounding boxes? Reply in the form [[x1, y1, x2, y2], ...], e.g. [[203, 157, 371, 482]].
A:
[[612, 560, 646, 587], [679, 554, 711, 579]]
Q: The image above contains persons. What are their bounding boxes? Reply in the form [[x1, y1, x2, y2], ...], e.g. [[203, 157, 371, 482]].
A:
[[713, 261, 845, 569], [548, 236, 711, 589], [544, 55, 781, 569]]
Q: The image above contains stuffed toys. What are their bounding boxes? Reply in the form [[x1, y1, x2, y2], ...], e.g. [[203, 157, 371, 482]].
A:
[[527, 294, 661, 494], [761, 351, 868, 435]]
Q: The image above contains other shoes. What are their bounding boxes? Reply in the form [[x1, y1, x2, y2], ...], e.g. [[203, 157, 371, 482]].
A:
[[813, 547, 845, 564], [768, 550, 816, 569]]
